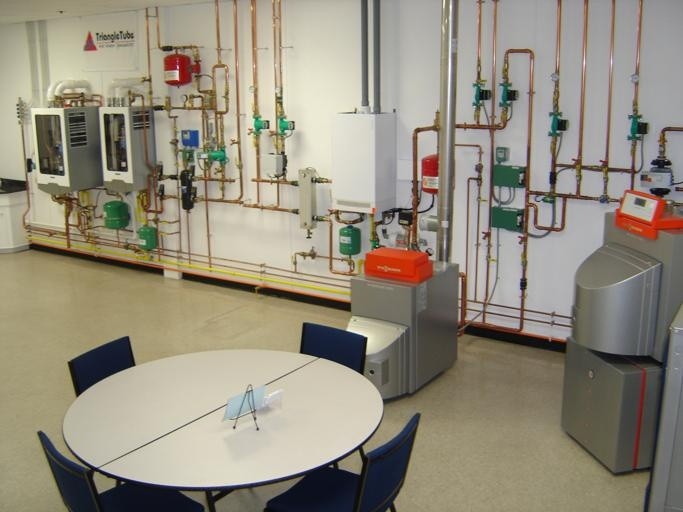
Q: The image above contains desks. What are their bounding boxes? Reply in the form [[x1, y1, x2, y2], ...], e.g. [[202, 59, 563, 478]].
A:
[[59, 348, 383, 491]]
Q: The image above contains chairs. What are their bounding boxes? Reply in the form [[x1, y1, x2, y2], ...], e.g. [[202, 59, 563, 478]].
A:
[[33, 335, 205, 511], [264, 320, 422, 511]]
